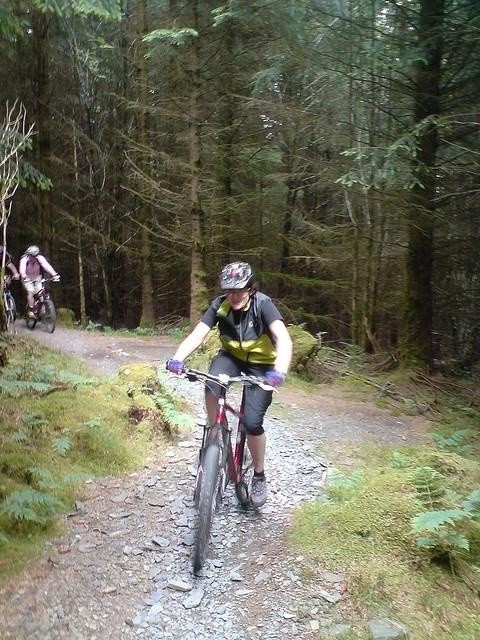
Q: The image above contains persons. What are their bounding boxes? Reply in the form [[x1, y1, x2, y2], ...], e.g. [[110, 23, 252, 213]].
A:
[[19, 244, 60, 320], [168, 261, 293, 507], [0, 245, 20, 280]]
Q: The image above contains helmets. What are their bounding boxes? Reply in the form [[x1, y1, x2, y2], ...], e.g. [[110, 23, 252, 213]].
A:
[[219, 261, 254, 291], [25, 245, 40, 256]]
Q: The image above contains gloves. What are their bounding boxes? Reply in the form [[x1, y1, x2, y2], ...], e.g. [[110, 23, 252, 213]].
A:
[[23, 276, 31, 282], [53, 273, 61, 281], [263, 370, 284, 387], [169, 359, 184, 376]]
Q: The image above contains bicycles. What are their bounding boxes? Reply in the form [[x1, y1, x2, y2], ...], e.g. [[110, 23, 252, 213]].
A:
[[3, 274, 61, 333], [165, 359, 285, 573]]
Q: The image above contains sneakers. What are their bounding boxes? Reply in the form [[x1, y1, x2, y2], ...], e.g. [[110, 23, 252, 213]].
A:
[[27, 311, 36, 319], [250, 476, 267, 507]]
